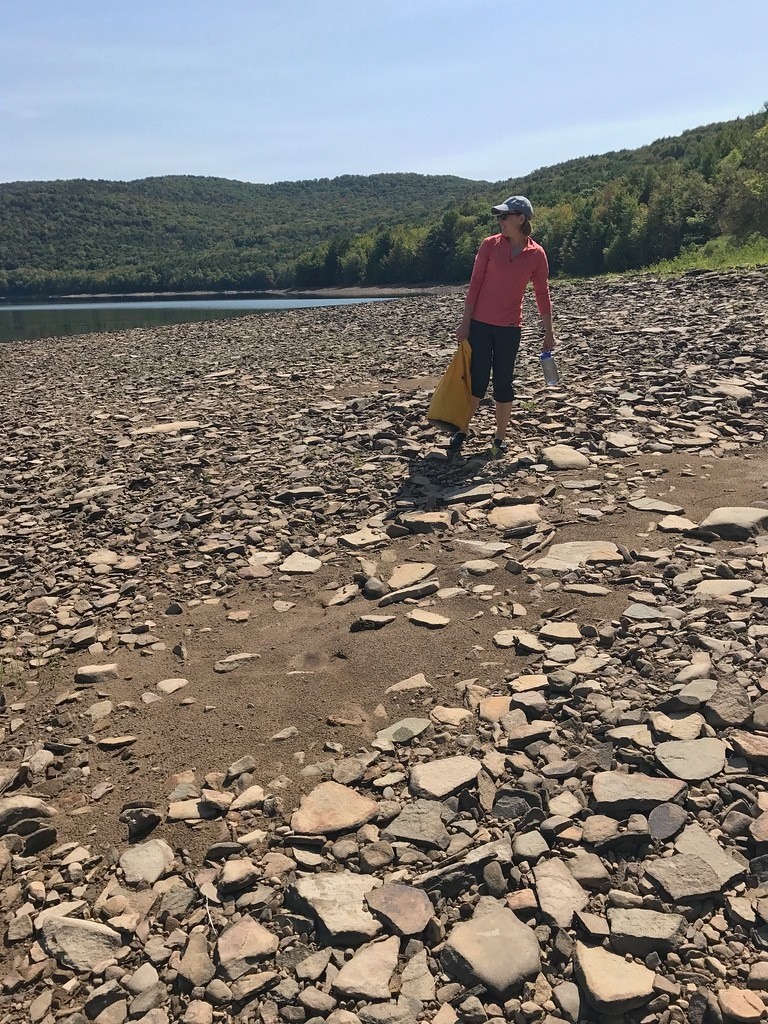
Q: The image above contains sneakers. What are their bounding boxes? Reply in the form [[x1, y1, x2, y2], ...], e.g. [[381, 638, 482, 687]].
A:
[[493, 438, 509, 453], [447, 447, 467, 466]]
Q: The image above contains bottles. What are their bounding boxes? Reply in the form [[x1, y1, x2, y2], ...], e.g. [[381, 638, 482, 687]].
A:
[[540, 350, 559, 385]]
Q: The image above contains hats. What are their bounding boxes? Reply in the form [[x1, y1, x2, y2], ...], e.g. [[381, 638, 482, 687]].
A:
[[491, 196, 534, 220]]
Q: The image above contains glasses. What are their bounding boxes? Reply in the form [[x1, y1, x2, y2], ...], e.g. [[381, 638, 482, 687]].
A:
[[497, 214, 519, 221]]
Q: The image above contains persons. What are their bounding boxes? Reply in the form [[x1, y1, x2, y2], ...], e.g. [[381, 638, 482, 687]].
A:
[[446, 196, 557, 466]]
